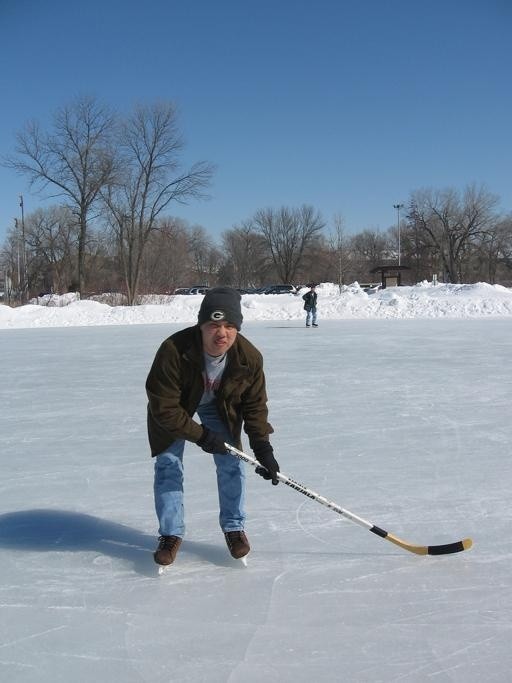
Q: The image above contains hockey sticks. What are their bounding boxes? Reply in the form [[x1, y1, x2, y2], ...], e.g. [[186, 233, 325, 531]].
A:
[[224, 441, 473, 556]]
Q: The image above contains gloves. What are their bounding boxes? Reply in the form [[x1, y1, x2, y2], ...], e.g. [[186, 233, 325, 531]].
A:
[[195, 423, 226, 454], [252, 452, 280, 485]]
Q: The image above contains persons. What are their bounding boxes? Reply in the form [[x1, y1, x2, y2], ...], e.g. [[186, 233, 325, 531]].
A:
[[302, 286, 318, 328], [145, 287, 279, 575]]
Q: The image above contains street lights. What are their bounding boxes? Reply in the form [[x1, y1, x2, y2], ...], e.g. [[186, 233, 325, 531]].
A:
[[393, 203, 404, 265]]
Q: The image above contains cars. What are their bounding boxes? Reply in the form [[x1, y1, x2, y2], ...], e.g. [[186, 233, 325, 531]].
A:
[[168, 283, 297, 296]]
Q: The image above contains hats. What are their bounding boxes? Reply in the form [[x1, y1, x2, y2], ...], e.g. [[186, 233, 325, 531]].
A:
[[197, 286, 243, 330]]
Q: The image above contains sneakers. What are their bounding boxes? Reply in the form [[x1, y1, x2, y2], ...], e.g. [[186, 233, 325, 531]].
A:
[[153, 534, 181, 565], [306, 324, 319, 328], [224, 529, 251, 559]]
